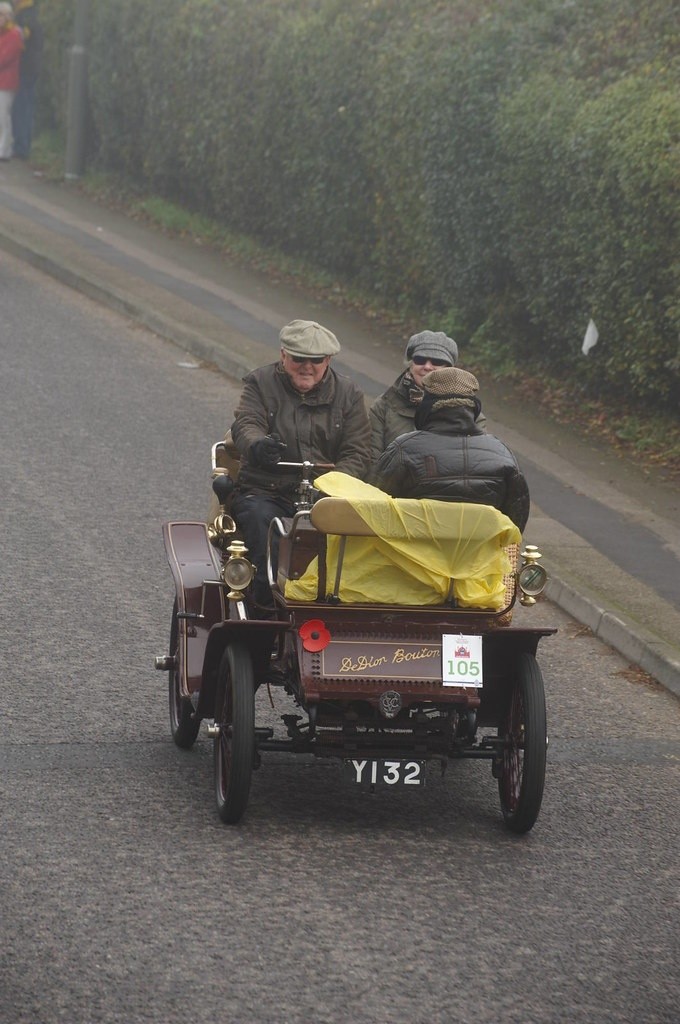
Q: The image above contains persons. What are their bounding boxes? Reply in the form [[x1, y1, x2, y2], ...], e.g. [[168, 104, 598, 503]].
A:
[[367, 330, 488, 489], [228, 320, 370, 616], [12, 1, 45, 162], [368, 367, 529, 542], [1, 1, 25, 163]]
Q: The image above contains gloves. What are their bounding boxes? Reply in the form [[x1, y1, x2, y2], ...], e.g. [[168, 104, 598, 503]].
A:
[[254, 433, 282, 466], [308, 490, 331, 505]]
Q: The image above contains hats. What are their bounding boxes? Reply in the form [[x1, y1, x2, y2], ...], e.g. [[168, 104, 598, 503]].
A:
[[279, 319, 340, 358], [421, 368, 479, 397], [406, 330, 459, 367]]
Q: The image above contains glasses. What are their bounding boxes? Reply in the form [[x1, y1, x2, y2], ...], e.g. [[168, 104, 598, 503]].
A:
[[413, 356, 445, 367], [290, 355, 325, 364]]
